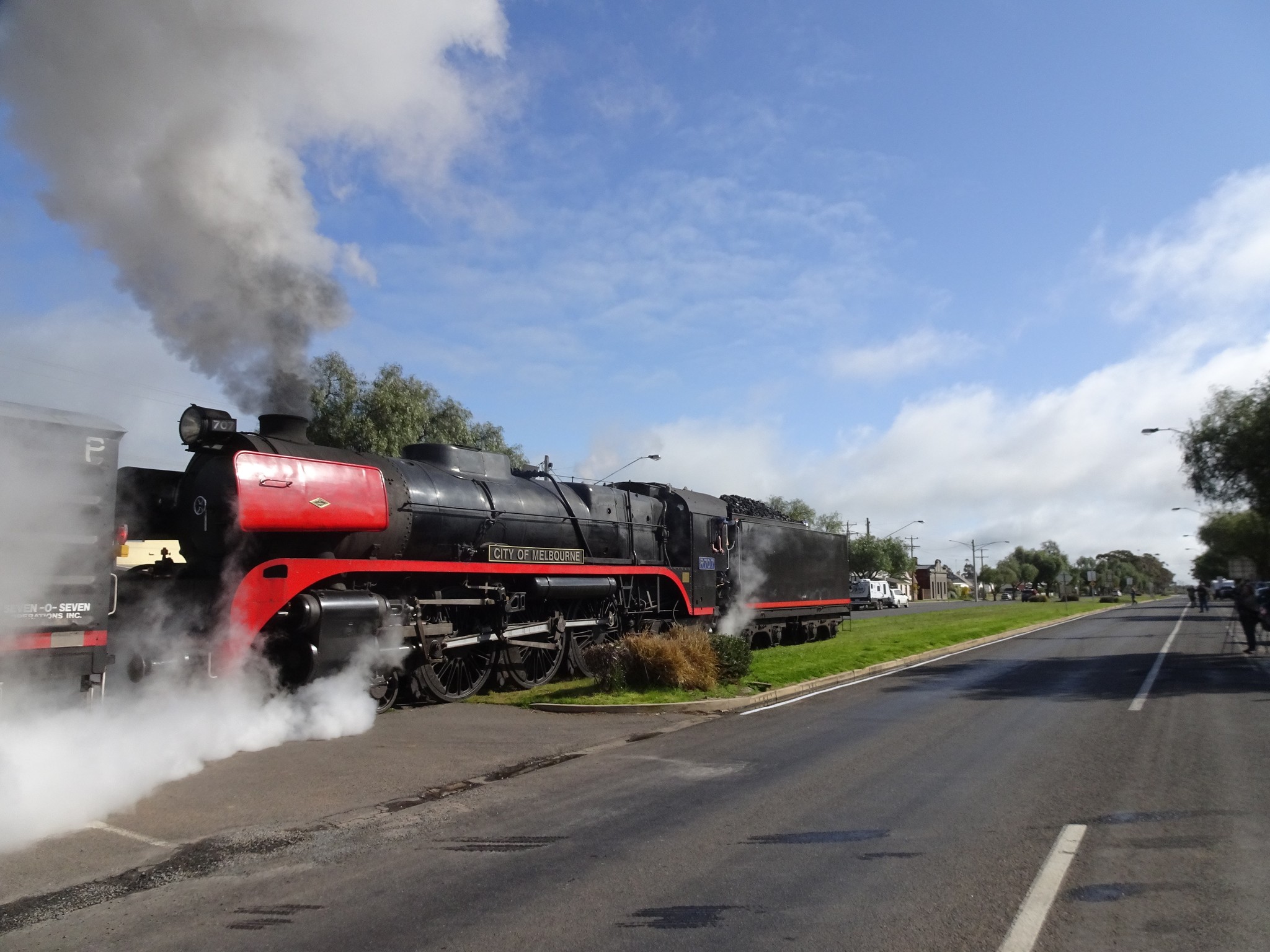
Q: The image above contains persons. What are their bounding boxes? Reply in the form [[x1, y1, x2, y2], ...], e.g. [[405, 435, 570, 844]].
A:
[[1229, 576, 1259, 653], [1187, 581, 1210, 614], [1131, 586, 1137, 605]]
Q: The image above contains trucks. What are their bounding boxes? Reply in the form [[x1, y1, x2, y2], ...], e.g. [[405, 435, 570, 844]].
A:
[[849, 579, 892, 611]]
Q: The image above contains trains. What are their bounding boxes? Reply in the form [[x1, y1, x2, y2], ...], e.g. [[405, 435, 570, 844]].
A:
[[0, 404, 853, 713]]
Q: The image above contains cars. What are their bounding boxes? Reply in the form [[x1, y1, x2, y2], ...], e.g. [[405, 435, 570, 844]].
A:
[[1001, 593, 1011, 600], [1111, 590, 1122, 597], [890, 588, 909, 608], [1217, 586, 1234, 600]]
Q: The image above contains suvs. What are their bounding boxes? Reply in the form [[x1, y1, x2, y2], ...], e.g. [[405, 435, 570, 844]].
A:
[[1021, 588, 1038, 602]]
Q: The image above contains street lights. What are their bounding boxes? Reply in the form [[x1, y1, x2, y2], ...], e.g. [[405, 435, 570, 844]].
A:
[[949, 539, 1010, 603], [842, 518, 925, 577]]
